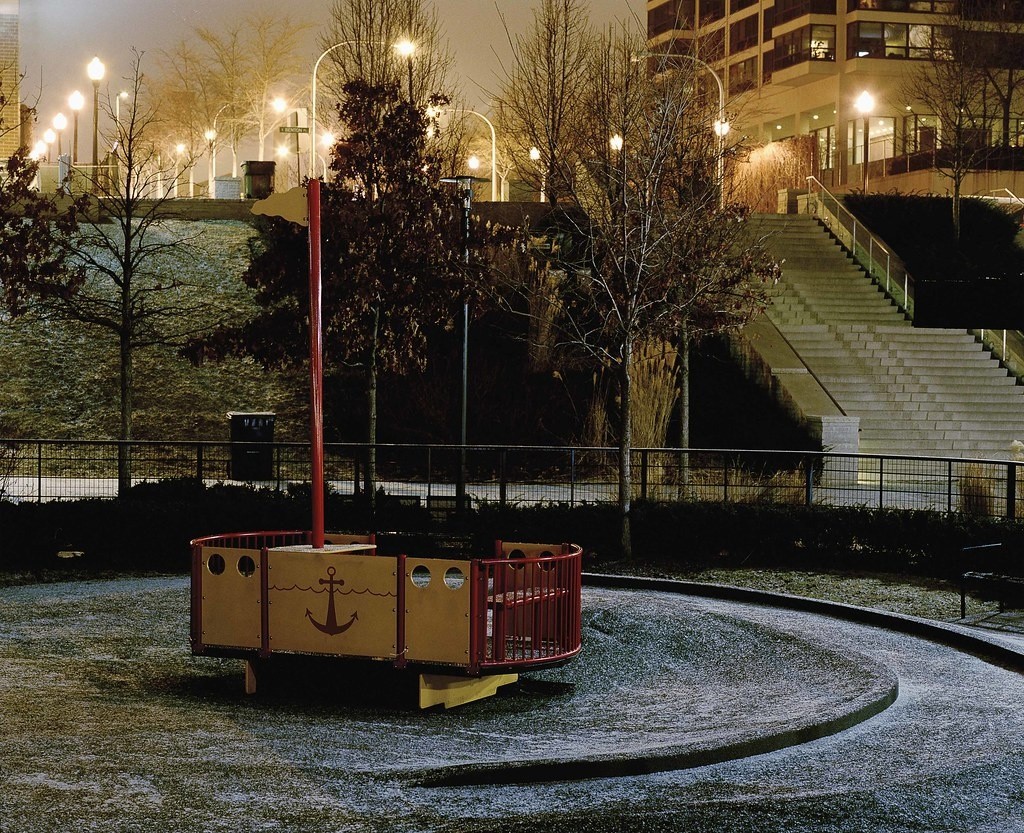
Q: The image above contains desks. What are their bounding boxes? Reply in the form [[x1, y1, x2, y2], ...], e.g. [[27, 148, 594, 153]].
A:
[[266, 544, 378, 556]]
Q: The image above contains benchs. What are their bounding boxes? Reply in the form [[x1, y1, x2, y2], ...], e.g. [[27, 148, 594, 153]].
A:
[[487, 587, 568, 662], [960, 543, 1024, 620]]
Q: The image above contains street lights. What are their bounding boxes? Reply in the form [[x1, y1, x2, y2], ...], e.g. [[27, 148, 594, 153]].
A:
[[426, 105, 500, 202], [24, 53, 129, 202], [440, 170, 492, 496], [141, 94, 335, 197], [627, 50, 725, 213], [309, 35, 419, 182], [853, 87, 874, 193], [459, 120, 729, 203]]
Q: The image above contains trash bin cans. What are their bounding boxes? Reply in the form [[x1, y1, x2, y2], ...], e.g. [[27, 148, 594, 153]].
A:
[[240, 160, 277, 200], [226, 410, 277, 483]]
